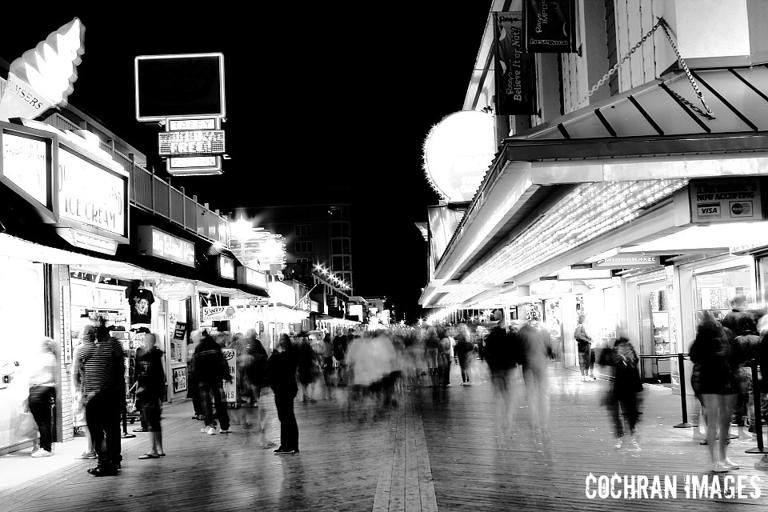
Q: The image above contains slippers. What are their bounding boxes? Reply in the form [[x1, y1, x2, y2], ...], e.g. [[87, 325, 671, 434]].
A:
[[138, 453, 165, 459]]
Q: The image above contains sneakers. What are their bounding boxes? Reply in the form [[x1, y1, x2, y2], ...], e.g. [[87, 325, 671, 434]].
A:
[[274, 449, 295, 454], [31, 448, 52, 457], [712, 462, 740, 472], [77, 451, 121, 476], [200, 425, 232, 435]]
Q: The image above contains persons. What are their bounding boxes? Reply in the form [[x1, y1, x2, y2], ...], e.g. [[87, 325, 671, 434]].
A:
[[28, 310, 739, 476]]
